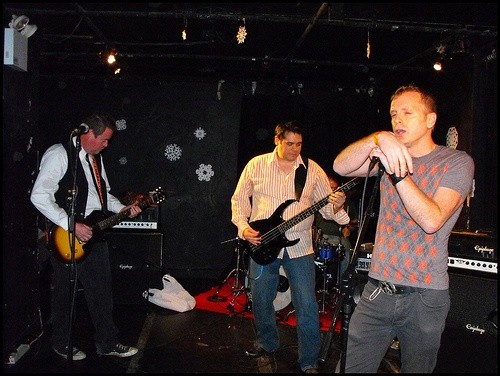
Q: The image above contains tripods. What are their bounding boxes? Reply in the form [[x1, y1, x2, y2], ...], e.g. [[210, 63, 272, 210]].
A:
[[316, 245, 333, 314], [212, 237, 247, 300]]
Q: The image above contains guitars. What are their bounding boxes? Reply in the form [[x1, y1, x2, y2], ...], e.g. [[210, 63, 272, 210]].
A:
[[241, 176, 365, 266], [50, 185, 168, 265]]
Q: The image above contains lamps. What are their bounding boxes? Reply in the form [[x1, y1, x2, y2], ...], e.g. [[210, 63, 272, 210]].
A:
[[210, 19, 248, 45], [3, 11, 38, 71], [432, 39, 464, 71]]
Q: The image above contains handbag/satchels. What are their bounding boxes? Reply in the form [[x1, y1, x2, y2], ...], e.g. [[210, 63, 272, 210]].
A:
[[144, 274, 196, 313]]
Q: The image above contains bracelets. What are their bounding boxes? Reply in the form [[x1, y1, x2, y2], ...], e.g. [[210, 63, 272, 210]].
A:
[[374, 131, 381, 145], [390, 170, 408, 185]]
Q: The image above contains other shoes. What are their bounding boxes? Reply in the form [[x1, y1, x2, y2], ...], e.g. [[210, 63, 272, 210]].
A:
[[256, 345, 278, 374], [305, 368, 319, 373]]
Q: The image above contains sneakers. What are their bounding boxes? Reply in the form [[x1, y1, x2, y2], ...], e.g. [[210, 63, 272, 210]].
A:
[[97, 343, 138, 357], [53, 343, 86, 361]]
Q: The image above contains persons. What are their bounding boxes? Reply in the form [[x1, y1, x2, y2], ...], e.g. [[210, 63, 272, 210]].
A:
[[332, 85, 475, 374], [30, 116, 141, 361], [314, 177, 350, 274], [231, 121, 350, 373]]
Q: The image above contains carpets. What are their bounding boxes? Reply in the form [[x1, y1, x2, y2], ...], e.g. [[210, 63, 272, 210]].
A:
[[191, 277, 345, 333]]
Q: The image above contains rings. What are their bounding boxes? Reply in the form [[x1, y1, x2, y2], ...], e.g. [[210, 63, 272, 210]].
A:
[[373, 148, 378, 151]]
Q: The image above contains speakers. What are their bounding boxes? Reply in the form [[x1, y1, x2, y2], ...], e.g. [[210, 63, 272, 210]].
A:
[[105, 231, 164, 289], [443, 270, 500, 344]]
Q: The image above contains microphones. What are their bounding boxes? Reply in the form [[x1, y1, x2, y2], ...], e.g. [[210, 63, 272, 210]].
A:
[[71, 123, 89, 135], [368, 156, 379, 172]]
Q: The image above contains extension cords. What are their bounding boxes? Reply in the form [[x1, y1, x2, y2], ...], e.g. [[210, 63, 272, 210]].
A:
[[9, 345, 29, 364]]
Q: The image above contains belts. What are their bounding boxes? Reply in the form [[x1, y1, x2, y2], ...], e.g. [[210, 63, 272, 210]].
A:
[[369, 275, 424, 295]]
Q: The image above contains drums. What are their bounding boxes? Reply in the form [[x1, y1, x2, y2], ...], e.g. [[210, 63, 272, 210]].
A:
[[311, 243, 346, 264], [243, 263, 292, 313]]
